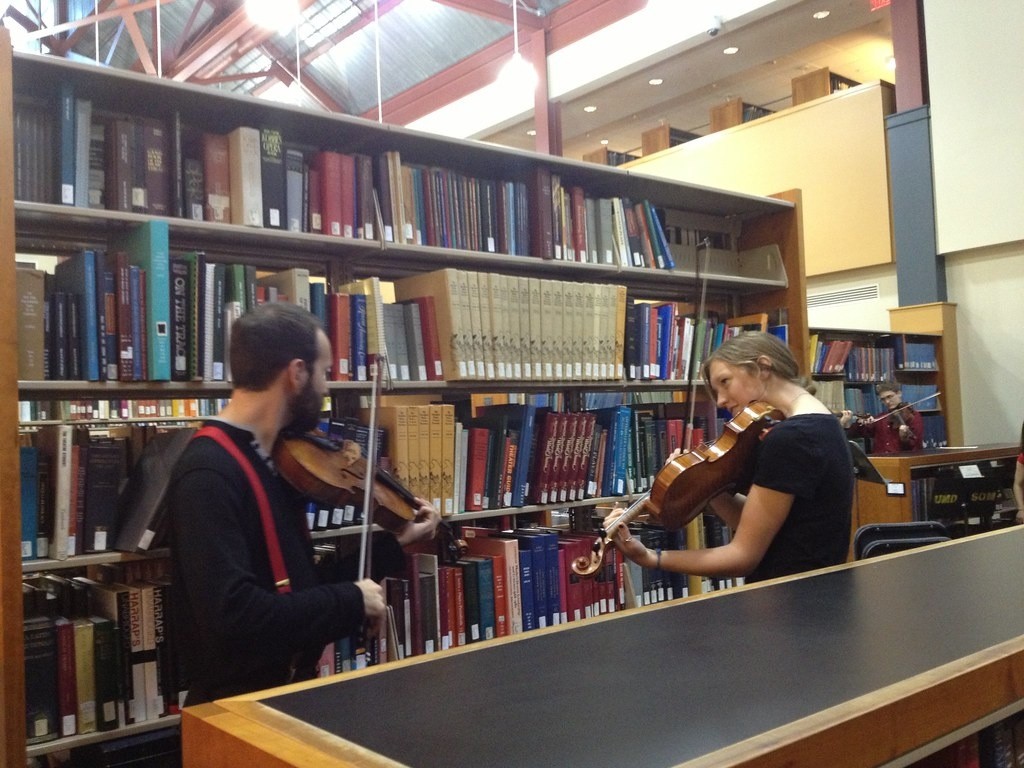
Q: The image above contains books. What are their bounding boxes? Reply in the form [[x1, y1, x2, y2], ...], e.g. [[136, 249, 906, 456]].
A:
[[909, 707, 1024, 767], [910, 458, 1012, 536], [811, 329, 949, 451], [828, 72, 855, 99], [12, 74, 798, 766]]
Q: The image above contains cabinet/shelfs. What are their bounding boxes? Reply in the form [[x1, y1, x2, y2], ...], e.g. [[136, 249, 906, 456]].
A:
[[583, 66, 864, 168], [846, 443, 1024, 564], [808, 326, 950, 456], [183, 524, 1023, 768], [0, 25, 814, 768]]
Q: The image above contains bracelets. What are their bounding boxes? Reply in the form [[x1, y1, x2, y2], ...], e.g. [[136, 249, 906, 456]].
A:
[[654, 548, 661, 571]]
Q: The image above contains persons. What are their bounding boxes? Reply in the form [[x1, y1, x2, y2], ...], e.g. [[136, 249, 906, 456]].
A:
[[602, 331, 855, 586], [156, 301, 442, 709], [1013, 420, 1024, 526], [838, 379, 924, 454]]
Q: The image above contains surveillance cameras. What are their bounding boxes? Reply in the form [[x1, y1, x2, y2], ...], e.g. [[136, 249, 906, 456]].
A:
[[705, 16, 721, 37]]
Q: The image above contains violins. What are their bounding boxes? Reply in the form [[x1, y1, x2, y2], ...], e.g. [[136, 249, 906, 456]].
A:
[[569, 397, 787, 577], [888, 402, 918, 443], [279, 430, 470, 557]]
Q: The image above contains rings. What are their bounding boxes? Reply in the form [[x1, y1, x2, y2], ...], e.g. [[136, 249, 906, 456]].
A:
[[624, 536, 632, 543]]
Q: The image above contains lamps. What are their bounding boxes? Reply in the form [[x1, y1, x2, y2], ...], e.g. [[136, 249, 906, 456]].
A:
[[496, 0, 539, 92]]
